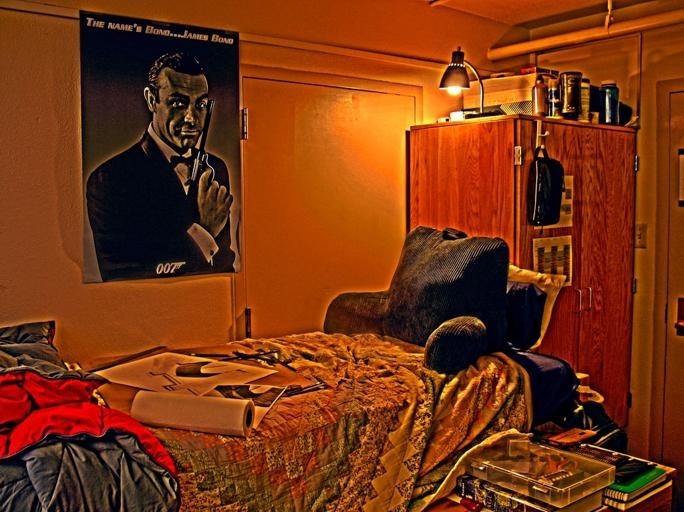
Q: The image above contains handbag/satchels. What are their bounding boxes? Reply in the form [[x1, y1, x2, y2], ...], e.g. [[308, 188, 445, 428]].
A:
[[566, 404, 627, 452], [527, 146, 564, 226]]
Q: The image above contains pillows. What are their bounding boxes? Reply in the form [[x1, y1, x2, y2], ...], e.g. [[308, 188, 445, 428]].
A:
[[324, 290, 388, 334], [422, 316, 489, 374], [381, 225, 510, 346], [509, 265, 567, 354]]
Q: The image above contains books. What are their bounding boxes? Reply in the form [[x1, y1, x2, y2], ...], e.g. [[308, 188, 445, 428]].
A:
[[603, 475, 677, 511], [565, 440, 668, 503]]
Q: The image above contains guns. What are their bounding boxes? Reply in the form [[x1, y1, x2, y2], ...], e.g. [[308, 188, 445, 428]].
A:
[[186, 95, 233, 218]]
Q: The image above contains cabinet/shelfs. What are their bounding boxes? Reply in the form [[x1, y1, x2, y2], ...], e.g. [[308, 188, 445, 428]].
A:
[[409, 123, 637, 427]]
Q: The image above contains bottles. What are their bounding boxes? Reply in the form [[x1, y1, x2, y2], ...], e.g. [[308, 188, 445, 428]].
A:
[[531, 72, 621, 125]]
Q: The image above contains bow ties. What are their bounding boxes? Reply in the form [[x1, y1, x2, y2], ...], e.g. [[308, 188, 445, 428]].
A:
[[170, 156, 194, 170]]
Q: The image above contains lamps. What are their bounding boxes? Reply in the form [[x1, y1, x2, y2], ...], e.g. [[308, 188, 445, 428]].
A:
[[440, 44, 504, 119]]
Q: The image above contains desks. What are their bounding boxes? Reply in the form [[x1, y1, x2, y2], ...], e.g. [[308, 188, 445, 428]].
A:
[[428, 481, 674, 511]]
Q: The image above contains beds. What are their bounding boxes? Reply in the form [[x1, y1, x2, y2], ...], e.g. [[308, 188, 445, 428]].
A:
[[1, 320, 582, 512]]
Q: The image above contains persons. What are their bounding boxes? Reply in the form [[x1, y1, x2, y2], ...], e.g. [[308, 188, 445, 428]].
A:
[[84, 50, 239, 282]]
